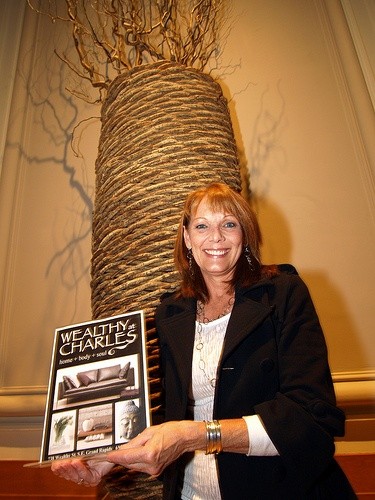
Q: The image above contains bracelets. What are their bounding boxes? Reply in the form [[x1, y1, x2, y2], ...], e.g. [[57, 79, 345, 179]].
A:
[[204, 419, 224, 457]]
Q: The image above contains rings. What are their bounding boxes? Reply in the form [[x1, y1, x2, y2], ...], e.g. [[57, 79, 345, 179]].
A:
[[76, 479, 83, 485]]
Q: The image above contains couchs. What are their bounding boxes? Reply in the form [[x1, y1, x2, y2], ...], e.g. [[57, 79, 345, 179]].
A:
[[58, 365, 135, 404]]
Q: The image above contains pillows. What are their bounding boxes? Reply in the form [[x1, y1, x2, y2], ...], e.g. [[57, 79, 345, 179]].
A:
[[77, 372, 91, 386], [121, 389, 139, 398], [118, 362, 131, 379], [63, 375, 82, 390]]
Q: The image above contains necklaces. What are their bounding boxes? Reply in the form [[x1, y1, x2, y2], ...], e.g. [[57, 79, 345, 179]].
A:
[[194, 294, 235, 389]]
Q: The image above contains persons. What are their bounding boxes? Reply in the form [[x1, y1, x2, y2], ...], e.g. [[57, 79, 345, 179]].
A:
[[52, 181, 358, 499]]
